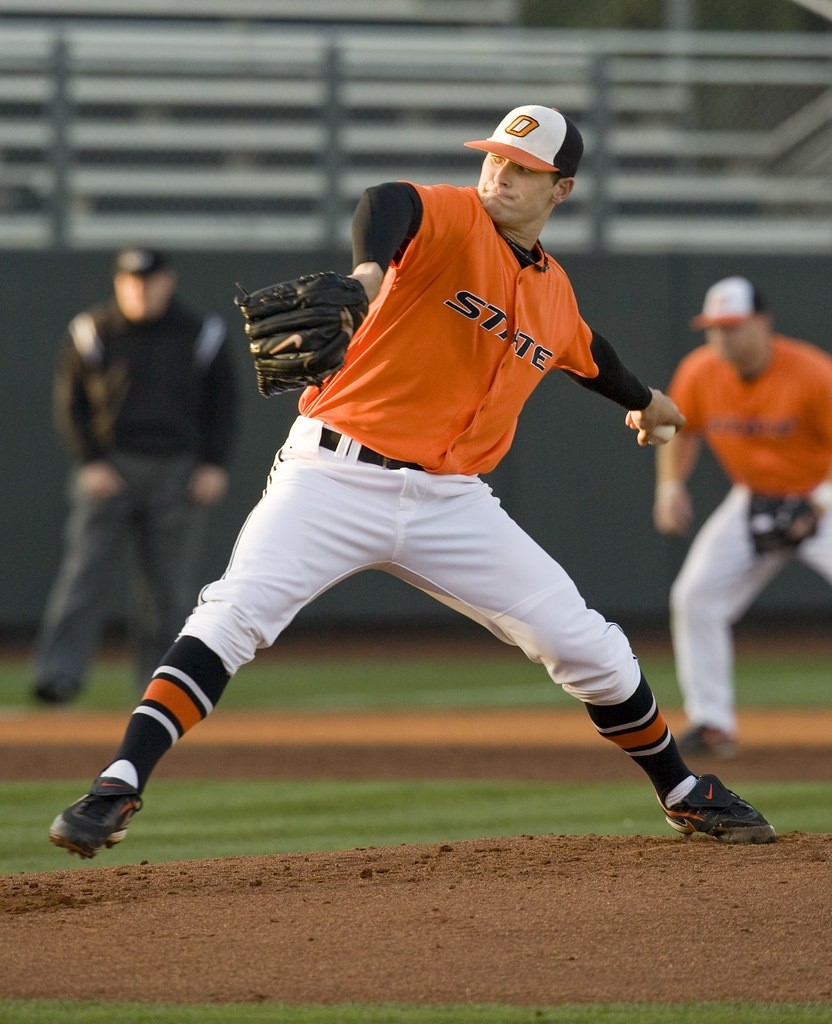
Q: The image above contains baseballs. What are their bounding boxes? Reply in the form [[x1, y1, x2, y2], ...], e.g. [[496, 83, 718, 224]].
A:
[[648, 424, 678, 447]]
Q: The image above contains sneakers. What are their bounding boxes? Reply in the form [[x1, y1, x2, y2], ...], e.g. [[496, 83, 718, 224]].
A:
[[656, 774, 776, 844], [676, 725, 737, 760], [49, 776, 144, 858]]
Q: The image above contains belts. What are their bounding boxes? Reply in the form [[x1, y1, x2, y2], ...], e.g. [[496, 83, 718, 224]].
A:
[[319, 427, 425, 471]]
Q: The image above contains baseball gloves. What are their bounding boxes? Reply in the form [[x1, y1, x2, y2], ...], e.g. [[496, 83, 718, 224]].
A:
[[236, 266, 372, 396]]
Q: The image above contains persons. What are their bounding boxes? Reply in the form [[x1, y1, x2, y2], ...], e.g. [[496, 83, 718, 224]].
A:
[[34, 247, 240, 704], [651, 277, 832, 757], [46, 102, 776, 860]]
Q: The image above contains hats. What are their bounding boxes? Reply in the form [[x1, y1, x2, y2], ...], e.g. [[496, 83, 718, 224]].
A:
[[691, 277, 769, 328], [464, 104, 584, 177]]
